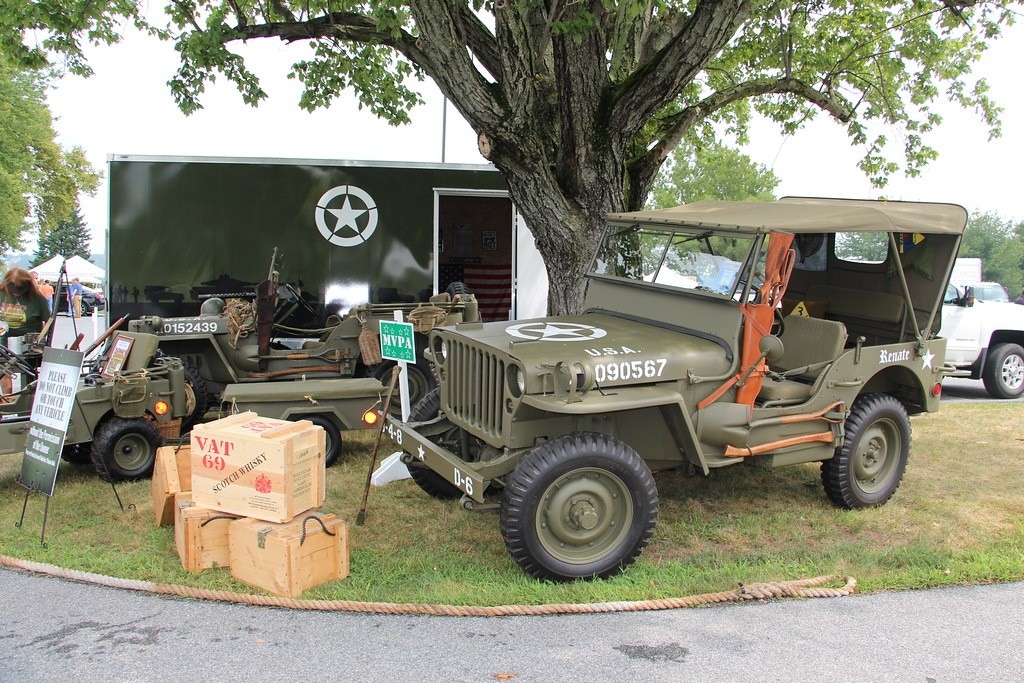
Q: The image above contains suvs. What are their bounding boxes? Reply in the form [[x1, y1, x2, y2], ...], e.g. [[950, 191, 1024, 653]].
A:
[[847, 260, 1024, 400], [375, 196, 970, 585], [126, 270, 485, 483], [0, 327, 187, 486]]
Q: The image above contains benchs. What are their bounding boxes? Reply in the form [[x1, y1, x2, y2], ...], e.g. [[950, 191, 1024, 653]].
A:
[[798, 284, 908, 348]]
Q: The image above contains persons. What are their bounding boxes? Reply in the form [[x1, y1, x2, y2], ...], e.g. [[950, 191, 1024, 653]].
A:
[[29, 271, 54, 314], [0, 268, 50, 395], [67, 278, 82, 318]]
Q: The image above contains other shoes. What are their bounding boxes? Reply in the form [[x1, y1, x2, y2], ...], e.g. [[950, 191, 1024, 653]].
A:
[[2, 413, 19, 419]]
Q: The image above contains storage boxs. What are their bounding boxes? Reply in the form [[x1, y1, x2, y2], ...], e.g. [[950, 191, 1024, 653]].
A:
[[190, 410, 327, 523], [174, 491, 247, 573], [228, 509, 350, 598], [152, 445, 192, 529]]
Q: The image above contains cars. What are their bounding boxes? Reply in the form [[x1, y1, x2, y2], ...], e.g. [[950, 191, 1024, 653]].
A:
[[966, 282, 1010, 303]]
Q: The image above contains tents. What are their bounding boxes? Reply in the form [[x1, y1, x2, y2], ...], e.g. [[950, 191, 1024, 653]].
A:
[[28, 255, 105, 281]]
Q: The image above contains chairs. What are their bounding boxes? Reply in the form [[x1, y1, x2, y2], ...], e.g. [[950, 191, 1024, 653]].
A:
[[302, 307, 358, 349], [756, 313, 848, 407]]
